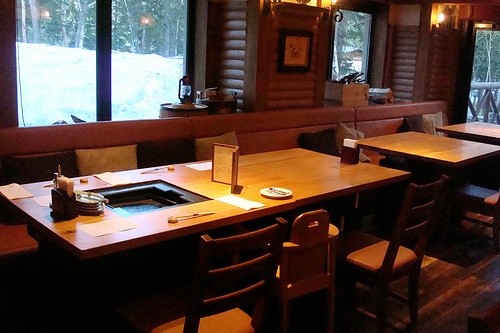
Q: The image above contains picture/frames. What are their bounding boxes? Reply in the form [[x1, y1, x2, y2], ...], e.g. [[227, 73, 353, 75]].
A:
[[276, 28, 315, 73]]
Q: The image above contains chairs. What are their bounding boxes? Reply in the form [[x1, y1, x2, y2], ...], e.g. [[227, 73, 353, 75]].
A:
[[336, 173, 449, 333], [448, 179, 500, 250], [113, 216, 285, 333], [272, 209, 340, 333]]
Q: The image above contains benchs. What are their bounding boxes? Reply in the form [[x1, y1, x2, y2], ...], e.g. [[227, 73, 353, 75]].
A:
[[0, 100, 450, 260]]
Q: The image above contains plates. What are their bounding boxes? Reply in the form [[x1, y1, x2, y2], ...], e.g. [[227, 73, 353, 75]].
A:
[[260, 187, 293, 198], [76, 192, 105, 214]]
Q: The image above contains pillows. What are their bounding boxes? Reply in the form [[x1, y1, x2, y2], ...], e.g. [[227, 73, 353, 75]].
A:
[[299, 121, 372, 163], [403, 111, 443, 137], [0, 131, 240, 226]]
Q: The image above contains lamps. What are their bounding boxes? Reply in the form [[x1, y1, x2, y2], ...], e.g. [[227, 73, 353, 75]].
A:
[[270, 0, 343, 23], [178, 75, 193, 103]]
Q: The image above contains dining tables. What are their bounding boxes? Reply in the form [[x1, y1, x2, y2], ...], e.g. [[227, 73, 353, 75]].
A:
[[0, 148, 411, 333]]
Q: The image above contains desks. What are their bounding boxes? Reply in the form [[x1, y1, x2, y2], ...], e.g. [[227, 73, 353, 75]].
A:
[[435, 121, 500, 144], [357, 131, 500, 169]]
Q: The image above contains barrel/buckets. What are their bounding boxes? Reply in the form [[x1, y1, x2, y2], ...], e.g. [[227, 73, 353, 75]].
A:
[[159, 103, 209, 118], [196, 100, 238, 115]]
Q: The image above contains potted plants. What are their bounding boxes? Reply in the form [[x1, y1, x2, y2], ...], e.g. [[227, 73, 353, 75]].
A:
[[324, 71, 369, 108]]
